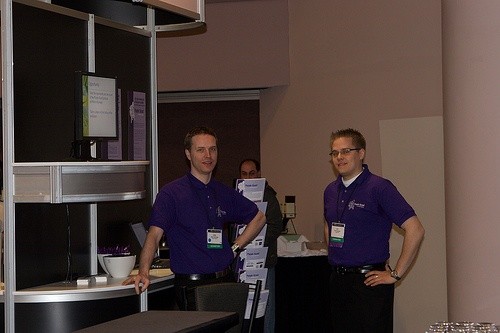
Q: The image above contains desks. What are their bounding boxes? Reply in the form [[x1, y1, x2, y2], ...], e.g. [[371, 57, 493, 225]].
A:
[[274, 249, 334, 333]]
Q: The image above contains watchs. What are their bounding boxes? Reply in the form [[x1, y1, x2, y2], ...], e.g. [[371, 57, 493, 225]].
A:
[[231, 243, 240, 258], [391, 270, 401, 281]]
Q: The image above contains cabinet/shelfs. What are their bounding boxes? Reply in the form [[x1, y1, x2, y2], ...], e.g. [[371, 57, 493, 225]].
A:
[[0, 0, 238, 333]]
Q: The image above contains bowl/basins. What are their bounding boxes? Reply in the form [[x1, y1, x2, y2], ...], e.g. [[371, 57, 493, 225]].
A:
[[97, 252, 130, 275], [104, 256, 136, 277]]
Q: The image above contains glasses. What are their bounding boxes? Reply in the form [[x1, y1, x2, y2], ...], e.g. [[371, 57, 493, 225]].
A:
[[329, 147, 361, 156]]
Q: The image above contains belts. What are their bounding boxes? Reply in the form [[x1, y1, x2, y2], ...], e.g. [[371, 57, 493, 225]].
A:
[[329, 264, 386, 275], [174, 266, 230, 282]]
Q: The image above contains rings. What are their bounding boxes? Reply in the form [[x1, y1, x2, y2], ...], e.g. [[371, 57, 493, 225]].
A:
[[376, 275, 378, 278]]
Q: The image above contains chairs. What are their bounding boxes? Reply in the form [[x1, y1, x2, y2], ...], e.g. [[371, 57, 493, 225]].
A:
[[180, 276, 264, 333]]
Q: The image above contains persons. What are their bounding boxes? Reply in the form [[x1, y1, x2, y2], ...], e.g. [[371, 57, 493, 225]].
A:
[[239, 158, 283, 333], [123, 125, 266, 333], [323, 129, 425, 333]]
